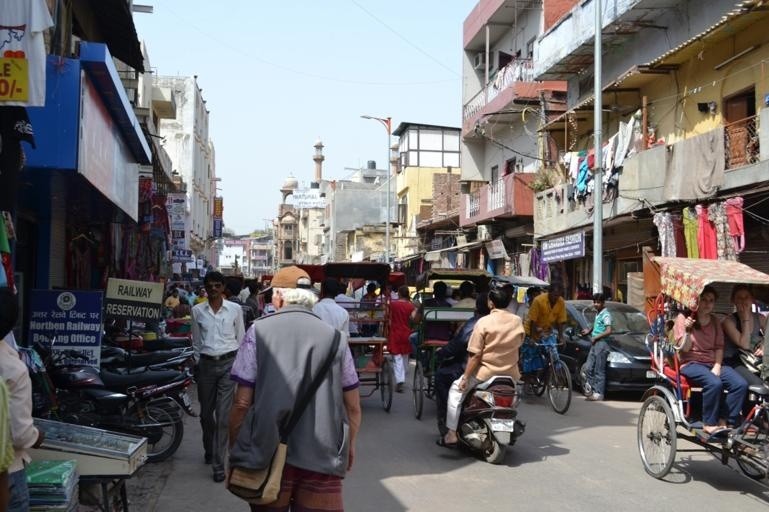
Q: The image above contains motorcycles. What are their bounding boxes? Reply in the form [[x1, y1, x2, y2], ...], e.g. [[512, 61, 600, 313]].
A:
[[24, 334, 203, 462], [434, 346, 527, 467]]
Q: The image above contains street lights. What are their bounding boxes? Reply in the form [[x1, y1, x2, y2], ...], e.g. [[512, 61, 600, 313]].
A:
[[359, 114, 393, 263]]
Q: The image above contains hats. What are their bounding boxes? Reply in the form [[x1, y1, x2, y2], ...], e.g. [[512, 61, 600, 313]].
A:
[[257, 266, 312, 294]]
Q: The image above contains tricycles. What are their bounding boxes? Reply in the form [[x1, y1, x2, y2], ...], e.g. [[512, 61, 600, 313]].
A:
[[318, 259, 395, 413], [408, 267, 491, 422], [492, 273, 574, 415], [635, 254, 769, 488]]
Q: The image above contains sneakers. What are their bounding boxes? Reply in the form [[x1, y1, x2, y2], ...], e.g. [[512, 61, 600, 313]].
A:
[[588, 393, 604, 401]]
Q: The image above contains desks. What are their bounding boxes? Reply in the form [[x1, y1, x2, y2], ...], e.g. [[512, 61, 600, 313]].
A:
[[79, 457, 150, 511]]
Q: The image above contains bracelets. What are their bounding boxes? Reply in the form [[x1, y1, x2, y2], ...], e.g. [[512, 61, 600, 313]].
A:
[[742, 320, 749, 323]]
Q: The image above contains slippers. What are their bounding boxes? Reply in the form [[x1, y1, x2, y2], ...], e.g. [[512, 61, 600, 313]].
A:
[[436, 435, 458, 449]]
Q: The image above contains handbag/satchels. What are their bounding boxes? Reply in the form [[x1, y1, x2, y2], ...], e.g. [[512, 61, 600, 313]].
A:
[[737, 347, 762, 373], [228, 441, 288, 505]]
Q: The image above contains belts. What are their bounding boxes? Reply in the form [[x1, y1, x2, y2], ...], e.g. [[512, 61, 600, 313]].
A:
[[200, 351, 237, 362]]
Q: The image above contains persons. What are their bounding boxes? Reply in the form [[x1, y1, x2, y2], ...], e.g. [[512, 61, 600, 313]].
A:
[[1, 378, 15, 512], [721, 284, 769, 419], [1, 286, 45, 512], [167, 265, 421, 512], [672, 286, 748, 435], [409, 275, 568, 371], [435, 289, 525, 450], [581, 293, 612, 400]]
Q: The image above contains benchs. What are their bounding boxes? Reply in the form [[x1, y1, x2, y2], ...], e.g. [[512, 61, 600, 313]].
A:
[[337, 300, 390, 373], [646, 307, 756, 417], [420, 306, 476, 371]]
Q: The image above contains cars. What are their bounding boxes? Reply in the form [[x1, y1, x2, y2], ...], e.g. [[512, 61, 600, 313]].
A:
[[550, 295, 668, 397]]
